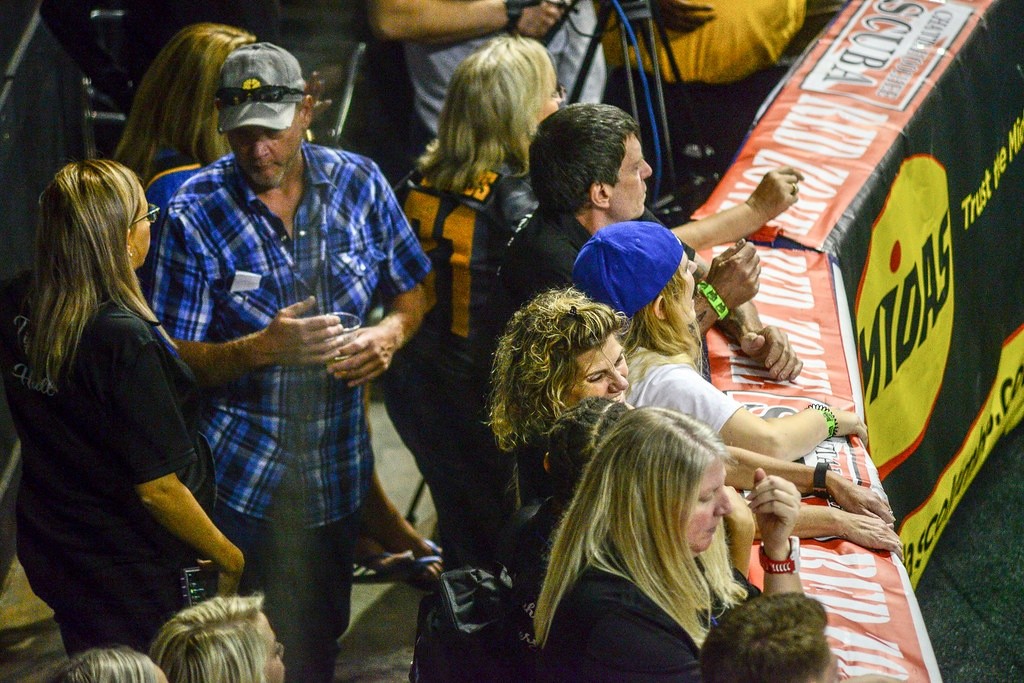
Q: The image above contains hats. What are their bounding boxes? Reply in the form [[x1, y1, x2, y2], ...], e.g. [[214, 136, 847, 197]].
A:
[[572, 220, 683, 322], [217, 41, 306, 133]]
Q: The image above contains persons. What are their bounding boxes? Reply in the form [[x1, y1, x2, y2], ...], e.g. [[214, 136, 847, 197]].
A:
[[0, 158, 245, 658], [111, 24, 435, 683], [44, 593, 285, 683], [367, 0, 902, 683]]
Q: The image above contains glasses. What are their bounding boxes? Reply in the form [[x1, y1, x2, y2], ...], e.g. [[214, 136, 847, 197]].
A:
[[550, 78, 568, 101], [215, 85, 306, 107], [130, 202, 160, 227]]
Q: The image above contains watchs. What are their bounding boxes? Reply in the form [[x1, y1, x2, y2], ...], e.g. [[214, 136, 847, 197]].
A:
[[759, 535, 802, 574]]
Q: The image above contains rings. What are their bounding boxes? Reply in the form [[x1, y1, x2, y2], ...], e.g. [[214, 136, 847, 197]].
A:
[[793, 184, 798, 196]]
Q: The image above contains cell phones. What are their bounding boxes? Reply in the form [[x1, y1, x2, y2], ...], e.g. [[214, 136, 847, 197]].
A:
[[180, 566, 209, 609]]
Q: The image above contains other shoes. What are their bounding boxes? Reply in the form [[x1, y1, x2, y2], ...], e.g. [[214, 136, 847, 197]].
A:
[[354, 536, 445, 593]]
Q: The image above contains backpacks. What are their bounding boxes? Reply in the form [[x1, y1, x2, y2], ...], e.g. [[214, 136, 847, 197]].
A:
[[408, 496, 701, 683]]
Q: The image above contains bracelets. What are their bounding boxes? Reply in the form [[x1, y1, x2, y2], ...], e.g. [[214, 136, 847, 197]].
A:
[[696, 280, 729, 320], [814, 462, 832, 500], [817, 410, 835, 439], [804, 404, 838, 439]]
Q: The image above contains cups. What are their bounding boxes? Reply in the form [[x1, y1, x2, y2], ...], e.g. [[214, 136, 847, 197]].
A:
[[326, 312, 362, 369]]
